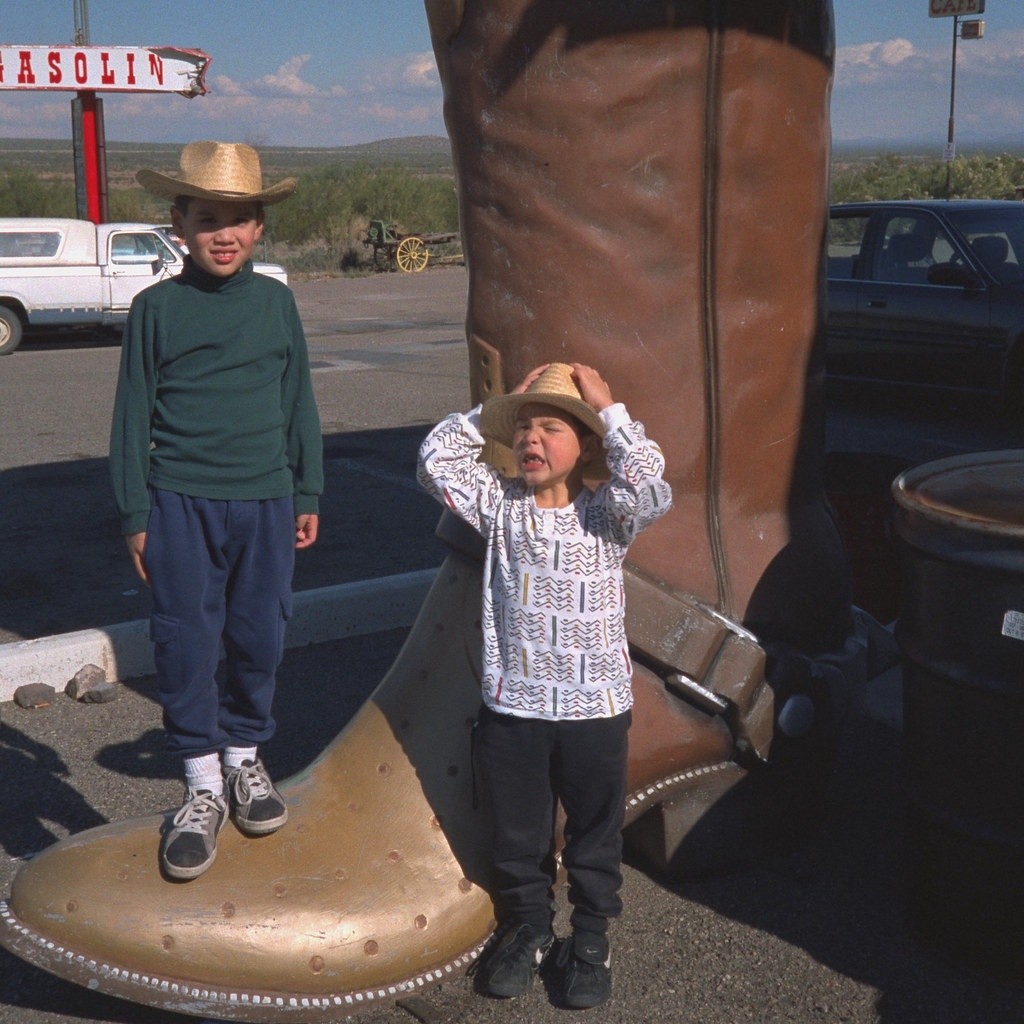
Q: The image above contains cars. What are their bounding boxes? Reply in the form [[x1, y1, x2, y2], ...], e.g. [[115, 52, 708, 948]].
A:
[[823, 199, 1024, 410]]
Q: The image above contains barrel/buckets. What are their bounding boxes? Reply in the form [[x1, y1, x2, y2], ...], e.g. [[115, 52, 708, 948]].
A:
[[888, 447, 1024, 991]]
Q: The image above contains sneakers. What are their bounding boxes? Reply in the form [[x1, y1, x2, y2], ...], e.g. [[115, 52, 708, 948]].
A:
[[221, 757, 290, 834], [484, 923, 570, 995], [162, 778, 230, 880], [555, 925, 617, 1009]]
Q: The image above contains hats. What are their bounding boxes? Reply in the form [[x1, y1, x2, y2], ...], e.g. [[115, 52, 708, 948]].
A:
[[134, 139, 301, 206], [479, 363, 607, 447]]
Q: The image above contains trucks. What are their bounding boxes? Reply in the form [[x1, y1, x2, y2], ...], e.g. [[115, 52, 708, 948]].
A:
[[0, 216, 287, 355]]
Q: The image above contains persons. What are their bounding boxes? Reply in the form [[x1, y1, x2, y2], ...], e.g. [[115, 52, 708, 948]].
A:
[[108, 140, 325, 881], [418, 360, 672, 1009]]
